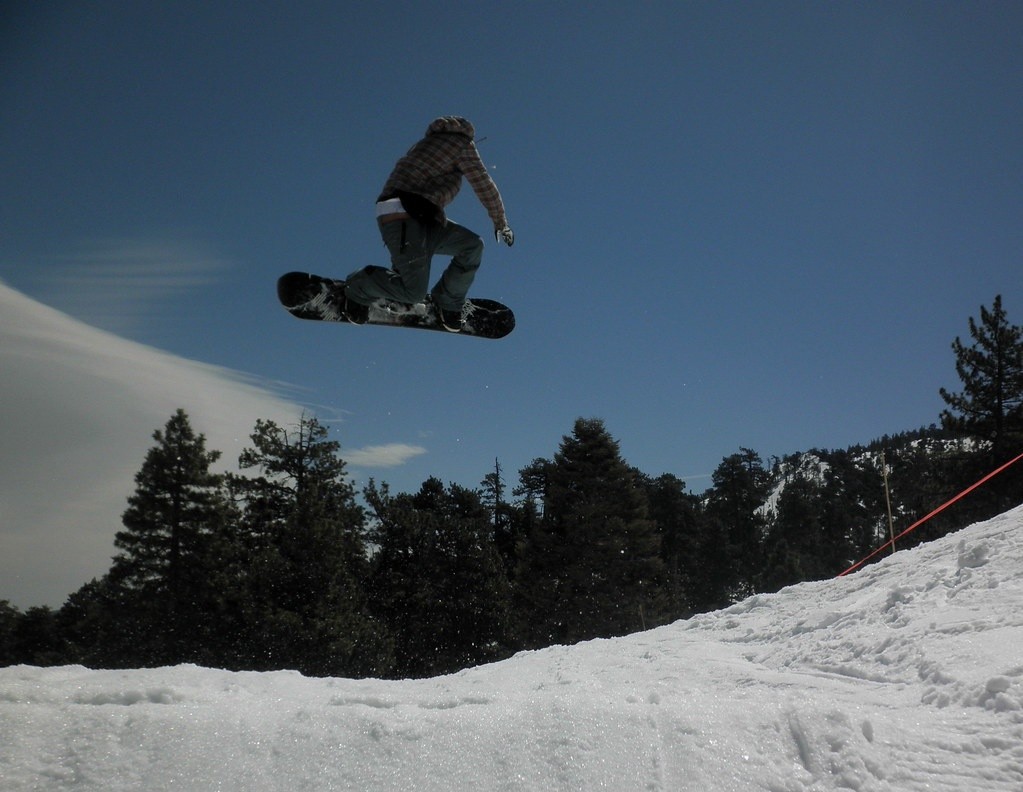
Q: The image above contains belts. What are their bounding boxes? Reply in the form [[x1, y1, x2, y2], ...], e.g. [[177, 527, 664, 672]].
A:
[[376, 213, 410, 226]]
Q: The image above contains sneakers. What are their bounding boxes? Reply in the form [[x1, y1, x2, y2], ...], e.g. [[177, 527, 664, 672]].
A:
[[346, 295, 370, 325], [441, 308, 462, 332]]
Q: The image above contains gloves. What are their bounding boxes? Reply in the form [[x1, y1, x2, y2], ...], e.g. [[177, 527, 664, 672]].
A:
[[494, 225, 514, 247]]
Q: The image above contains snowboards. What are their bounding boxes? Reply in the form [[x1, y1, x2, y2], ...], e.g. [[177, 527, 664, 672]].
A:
[[277, 272, 518, 339]]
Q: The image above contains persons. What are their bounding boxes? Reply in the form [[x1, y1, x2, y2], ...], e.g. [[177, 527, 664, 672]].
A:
[[342, 116, 513, 333]]
